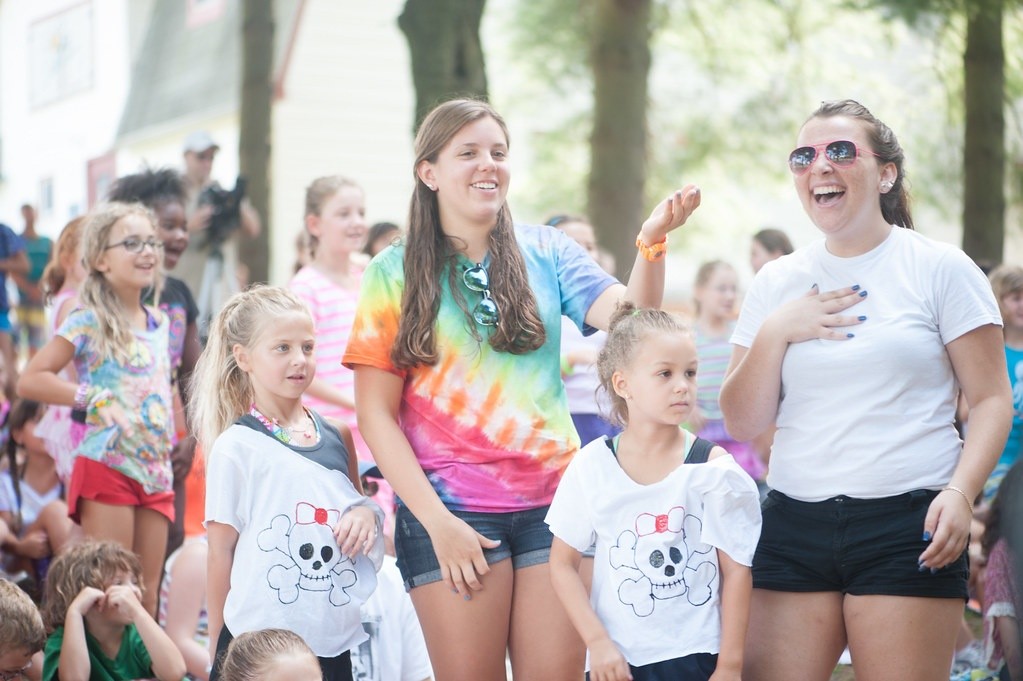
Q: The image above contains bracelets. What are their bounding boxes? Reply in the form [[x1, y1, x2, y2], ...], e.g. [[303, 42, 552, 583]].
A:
[[82, 386, 102, 402], [73, 381, 89, 411], [90, 394, 115, 421], [85, 388, 110, 407], [942, 487, 974, 514]]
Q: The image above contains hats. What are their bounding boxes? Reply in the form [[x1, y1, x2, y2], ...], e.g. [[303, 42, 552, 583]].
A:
[[183, 134, 221, 154], [358, 461, 384, 479]]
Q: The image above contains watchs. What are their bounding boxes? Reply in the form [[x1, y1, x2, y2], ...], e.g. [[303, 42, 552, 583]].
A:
[[635, 235, 669, 261]]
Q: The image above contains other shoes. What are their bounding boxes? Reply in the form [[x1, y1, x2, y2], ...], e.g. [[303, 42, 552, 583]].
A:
[[949, 640, 1007, 681]]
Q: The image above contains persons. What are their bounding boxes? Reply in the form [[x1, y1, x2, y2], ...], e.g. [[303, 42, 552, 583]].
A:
[[720, 99, 1014, 681], [0, 134, 1023, 681], [344, 98, 700, 681]]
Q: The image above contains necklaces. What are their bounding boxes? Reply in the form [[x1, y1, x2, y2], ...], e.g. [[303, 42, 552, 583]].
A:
[[256, 407, 312, 439]]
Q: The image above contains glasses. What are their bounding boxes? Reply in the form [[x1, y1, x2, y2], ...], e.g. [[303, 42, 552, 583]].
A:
[[463, 261, 501, 328], [787, 138, 880, 176], [363, 481, 379, 496], [104, 234, 157, 255]]
